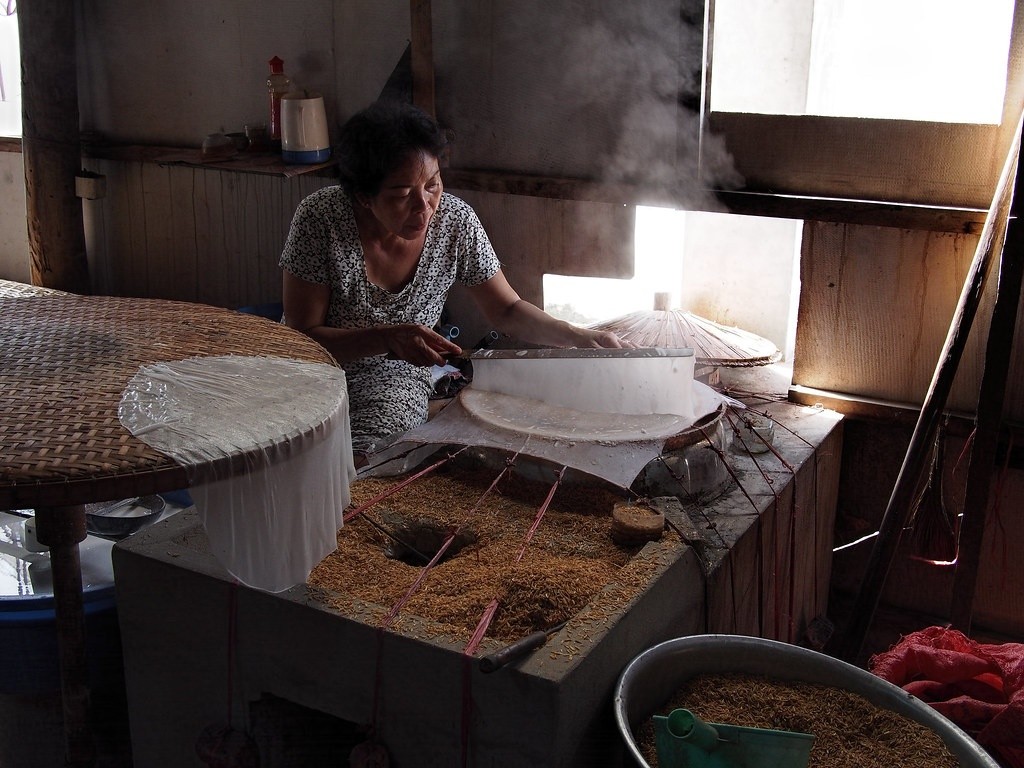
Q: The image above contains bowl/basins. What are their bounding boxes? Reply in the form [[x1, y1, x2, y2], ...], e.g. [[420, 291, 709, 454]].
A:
[[612, 632, 1001, 768]]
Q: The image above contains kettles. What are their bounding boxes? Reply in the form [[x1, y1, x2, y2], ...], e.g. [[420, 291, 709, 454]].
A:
[[279, 91, 331, 163]]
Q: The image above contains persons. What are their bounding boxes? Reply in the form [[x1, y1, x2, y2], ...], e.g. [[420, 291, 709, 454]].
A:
[[278, 98, 644, 469]]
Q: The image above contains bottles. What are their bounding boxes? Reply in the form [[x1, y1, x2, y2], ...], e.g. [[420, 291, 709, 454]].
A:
[[267, 56, 291, 143]]
